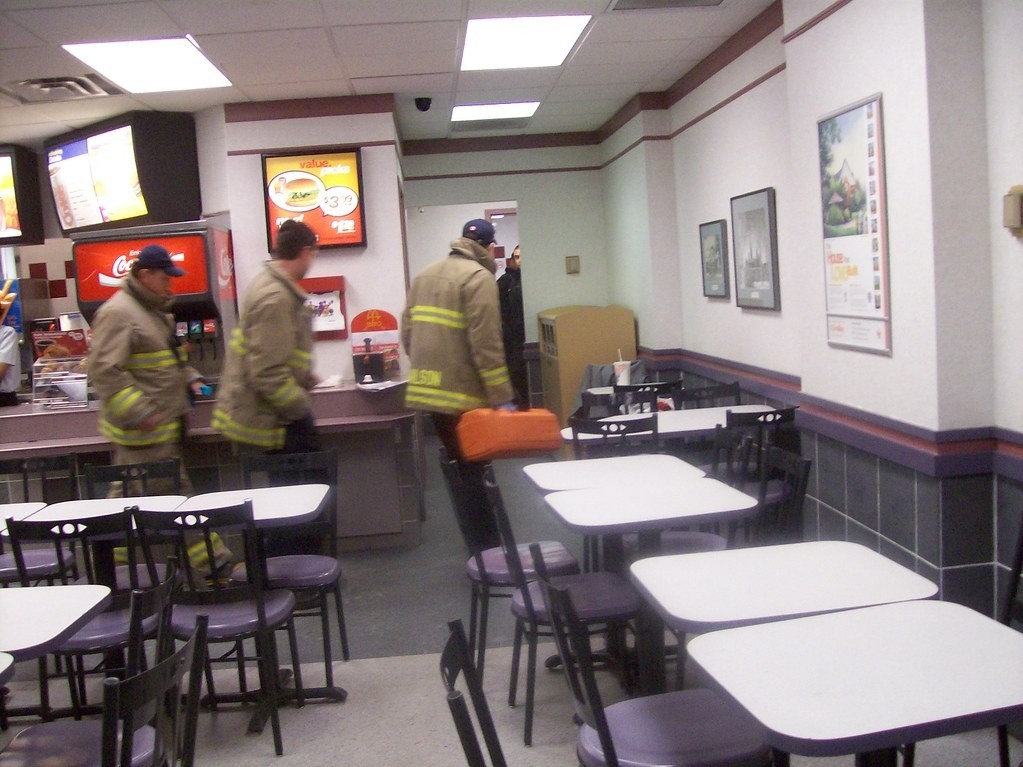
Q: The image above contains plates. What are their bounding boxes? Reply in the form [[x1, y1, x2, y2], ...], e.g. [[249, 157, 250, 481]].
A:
[[267, 170, 326, 212], [319, 185, 359, 217]]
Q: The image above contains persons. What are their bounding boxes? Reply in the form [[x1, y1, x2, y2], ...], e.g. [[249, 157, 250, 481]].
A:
[[496, 245, 528, 405], [210, 220, 317, 557], [400, 218, 514, 555], [0, 308, 22, 407], [87, 244, 244, 587]]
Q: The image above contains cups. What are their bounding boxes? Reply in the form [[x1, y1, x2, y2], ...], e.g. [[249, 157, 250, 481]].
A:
[[613, 361, 632, 387]]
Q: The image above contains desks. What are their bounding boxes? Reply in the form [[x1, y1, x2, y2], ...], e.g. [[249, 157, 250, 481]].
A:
[[174, 482, 348, 736], [0, 585, 113, 664], [0, 503, 54, 717], [543, 477, 759, 724], [685, 599, 1023, 767], [560, 404, 783, 572], [630, 540, 942, 634], [520, 455, 708, 689], [1, 494, 187, 724]]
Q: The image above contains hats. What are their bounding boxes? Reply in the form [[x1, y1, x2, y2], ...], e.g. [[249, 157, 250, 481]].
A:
[[134, 244, 187, 277], [463, 219, 498, 246]]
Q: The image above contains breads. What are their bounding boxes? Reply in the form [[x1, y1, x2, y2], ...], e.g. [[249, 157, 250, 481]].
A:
[[40, 345, 87, 374]]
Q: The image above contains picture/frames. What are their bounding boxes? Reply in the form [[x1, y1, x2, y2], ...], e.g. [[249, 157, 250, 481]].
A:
[[730, 187, 781, 312], [699, 219, 730, 299]]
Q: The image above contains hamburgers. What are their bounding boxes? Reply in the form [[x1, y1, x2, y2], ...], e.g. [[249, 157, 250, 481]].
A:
[[285, 178, 319, 207]]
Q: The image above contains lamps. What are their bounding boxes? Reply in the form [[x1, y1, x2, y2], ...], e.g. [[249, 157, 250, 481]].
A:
[[567, 256, 580, 274], [1002, 185, 1023, 236], [491, 214, 505, 220]]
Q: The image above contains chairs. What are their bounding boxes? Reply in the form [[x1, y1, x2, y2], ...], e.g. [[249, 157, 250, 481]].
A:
[[0, 380, 811, 767]]
